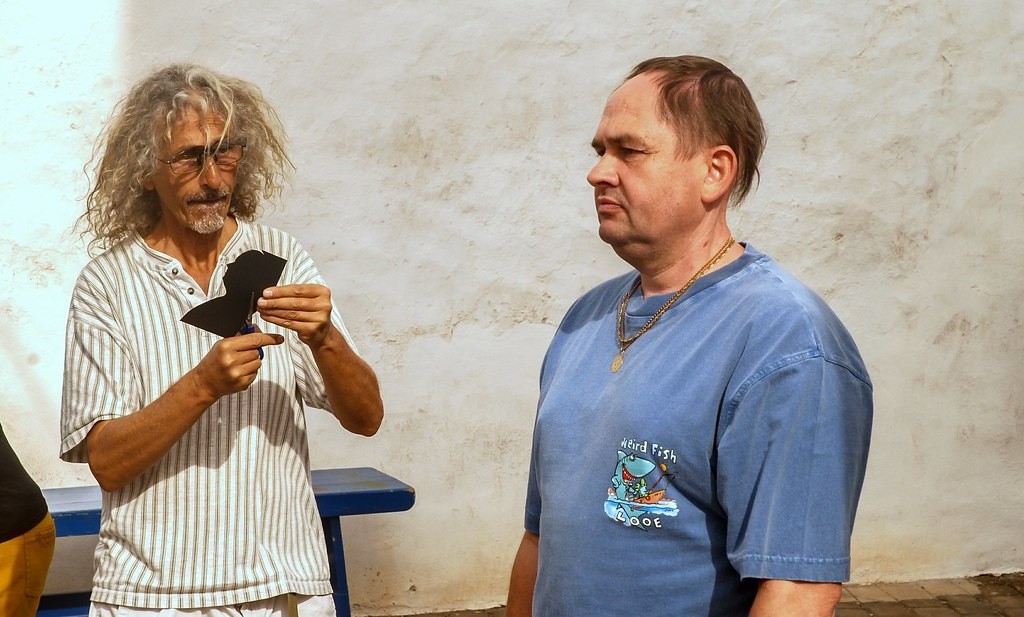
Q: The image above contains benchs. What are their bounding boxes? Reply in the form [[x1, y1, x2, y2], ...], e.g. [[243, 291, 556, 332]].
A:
[[36, 467, 414, 617]]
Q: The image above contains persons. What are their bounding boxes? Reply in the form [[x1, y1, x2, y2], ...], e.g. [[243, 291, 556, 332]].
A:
[[0, 423, 57, 617], [505, 54, 874, 617], [59, 64, 385, 617]]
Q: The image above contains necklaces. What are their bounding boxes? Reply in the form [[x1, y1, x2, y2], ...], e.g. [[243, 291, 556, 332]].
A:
[[610, 237, 734, 373]]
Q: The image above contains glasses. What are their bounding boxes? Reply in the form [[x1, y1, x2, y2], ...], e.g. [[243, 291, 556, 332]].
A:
[[157, 139, 246, 174]]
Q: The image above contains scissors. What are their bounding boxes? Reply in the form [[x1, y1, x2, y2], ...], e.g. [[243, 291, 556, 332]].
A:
[[235, 291, 264, 362]]
